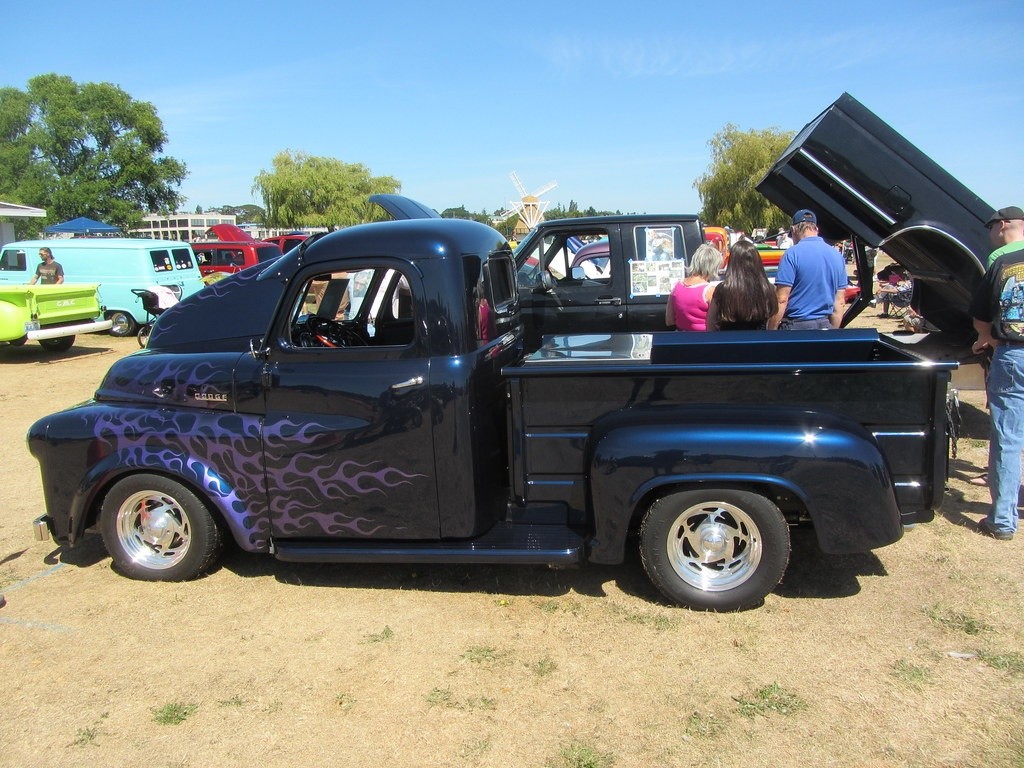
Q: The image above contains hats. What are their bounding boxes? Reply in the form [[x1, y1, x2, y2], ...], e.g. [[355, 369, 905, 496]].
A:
[[984, 206, 1024, 228], [787, 209, 817, 237]]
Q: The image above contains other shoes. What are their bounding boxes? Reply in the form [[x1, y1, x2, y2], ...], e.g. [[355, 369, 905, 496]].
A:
[[877, 313, 889, 318], [979, 518, 1013, 540], [869, 302, 876, 308]]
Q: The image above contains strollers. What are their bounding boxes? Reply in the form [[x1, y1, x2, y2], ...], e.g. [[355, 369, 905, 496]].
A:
[[130, 284, 185, 348]]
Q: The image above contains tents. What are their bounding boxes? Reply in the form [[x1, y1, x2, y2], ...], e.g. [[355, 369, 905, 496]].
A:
[[44, 217, 121, 239], [0, 202, 47, 217]]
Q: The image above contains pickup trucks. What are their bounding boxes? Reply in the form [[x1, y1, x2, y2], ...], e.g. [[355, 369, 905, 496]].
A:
[[0, 281, 113, 350], [25, 214, 967, 614], [504, 90, 999, 369]]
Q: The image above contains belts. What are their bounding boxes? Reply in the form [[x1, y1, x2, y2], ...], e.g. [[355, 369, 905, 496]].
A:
[[998, 339, 1024, 347]]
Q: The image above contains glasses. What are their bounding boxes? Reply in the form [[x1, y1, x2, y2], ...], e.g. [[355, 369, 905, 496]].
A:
[[39, 252, 47, 256], [988, 220, 1011, 231]]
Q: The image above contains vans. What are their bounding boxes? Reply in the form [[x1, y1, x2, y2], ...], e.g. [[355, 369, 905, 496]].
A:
[[0, 237, 205, 338], [188, 241, 284, 291]]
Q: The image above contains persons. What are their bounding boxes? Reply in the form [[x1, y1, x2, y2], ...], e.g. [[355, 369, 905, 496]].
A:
[[971, 206, 1024, 542], [347, 266, 411, 345], [769, 209, 847, 330], [30, 247, 64, 285], [706, 240, 778, 332], [664, 243, 723, 332], [777, 227, 794, 250], [476, 281, 498, 345], [850, 235, 943, 332]]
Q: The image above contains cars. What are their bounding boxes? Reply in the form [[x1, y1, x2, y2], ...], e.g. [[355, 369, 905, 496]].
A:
[[204, 222, 312, 257]]
